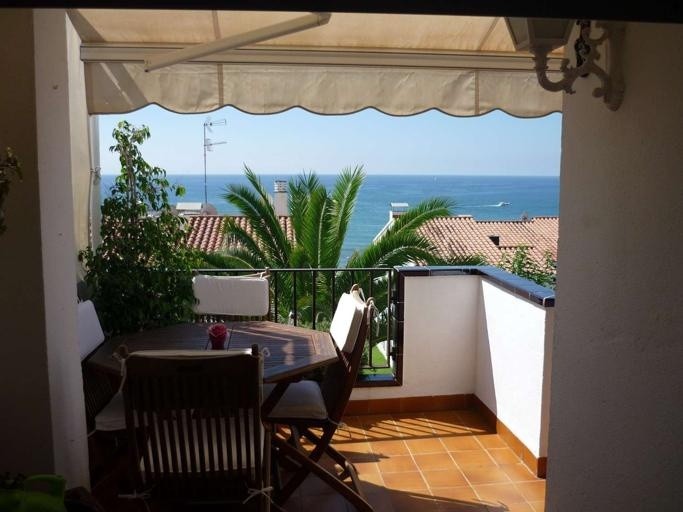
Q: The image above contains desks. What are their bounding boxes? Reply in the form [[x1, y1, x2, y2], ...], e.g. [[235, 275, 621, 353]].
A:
[[85, 319, 338, 422]]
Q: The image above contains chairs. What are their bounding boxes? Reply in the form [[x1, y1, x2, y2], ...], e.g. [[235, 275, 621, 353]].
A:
[[112, 344, 265, 512], [263, 285, 375, 512], [77, 299, 157, 431], [190, 267, 271, 323]]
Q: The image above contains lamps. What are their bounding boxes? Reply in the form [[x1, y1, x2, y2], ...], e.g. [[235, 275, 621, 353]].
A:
[[504, 17, 625, 111]]
